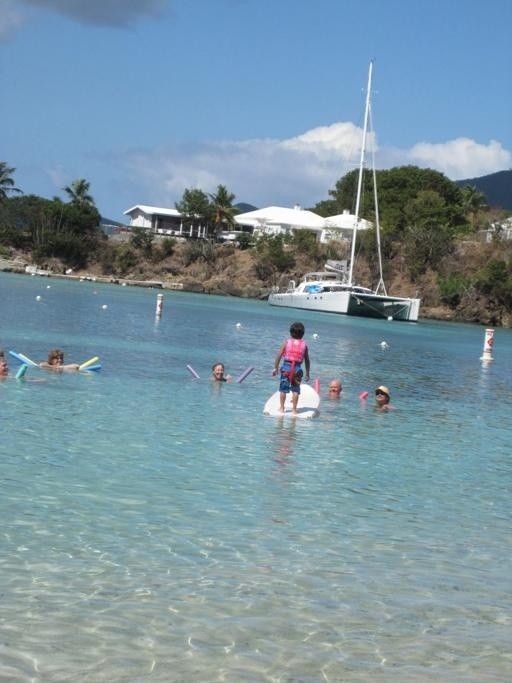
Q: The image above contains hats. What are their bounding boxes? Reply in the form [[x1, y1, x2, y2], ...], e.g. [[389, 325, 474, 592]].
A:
[[376, 386, 389, 397]]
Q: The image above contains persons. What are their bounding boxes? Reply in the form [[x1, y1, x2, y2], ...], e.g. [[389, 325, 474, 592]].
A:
[[272, 323, 310, 416], [40, 349, 81, 371], [328, 378, 342, 396], [0, 349, 10, 379], [273, 417, 296, 475], [207, 363, 231, 383], [374, 386, 394, 412]]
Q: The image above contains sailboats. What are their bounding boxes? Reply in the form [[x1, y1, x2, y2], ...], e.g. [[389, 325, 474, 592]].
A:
[[267, 58, 421, 323]]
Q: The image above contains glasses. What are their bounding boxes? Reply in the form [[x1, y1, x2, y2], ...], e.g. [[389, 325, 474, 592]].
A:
[[377, 392, 384, 395]]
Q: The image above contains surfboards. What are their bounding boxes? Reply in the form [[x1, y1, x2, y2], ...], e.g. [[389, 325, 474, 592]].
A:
[[263, 384, 320, 420]]
